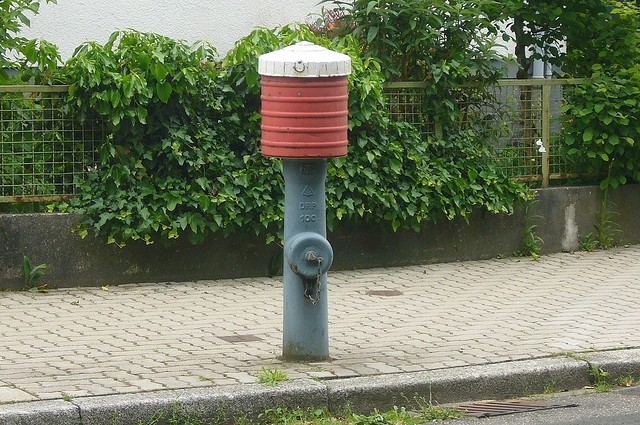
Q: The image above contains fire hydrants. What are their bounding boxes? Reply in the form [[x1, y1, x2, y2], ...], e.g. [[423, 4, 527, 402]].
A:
[[258, 41, 351, 360]]
